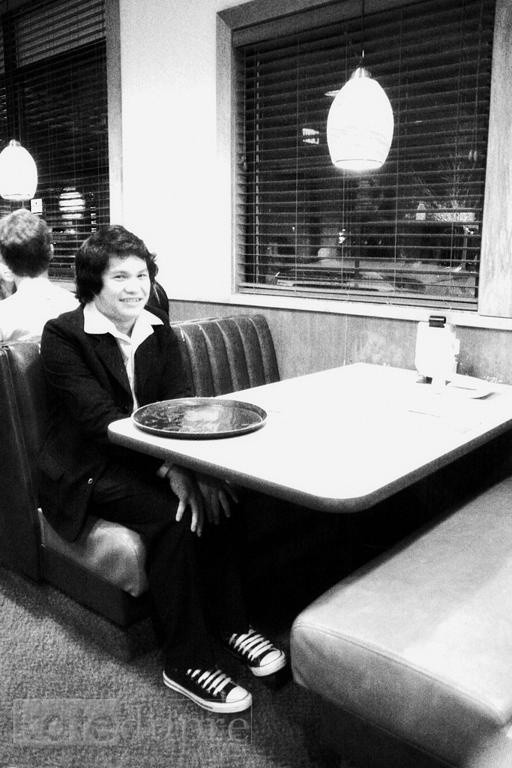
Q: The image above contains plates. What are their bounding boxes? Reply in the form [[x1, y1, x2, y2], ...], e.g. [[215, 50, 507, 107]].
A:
[[134, 397, 265, 440]]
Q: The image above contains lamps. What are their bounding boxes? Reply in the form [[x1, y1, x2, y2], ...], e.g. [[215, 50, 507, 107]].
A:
[[0, 134, 38, 201], [325, 49, 395, 172]]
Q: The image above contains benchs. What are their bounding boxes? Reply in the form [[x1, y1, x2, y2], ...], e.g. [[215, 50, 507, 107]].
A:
[[0, 315, 512, 768]]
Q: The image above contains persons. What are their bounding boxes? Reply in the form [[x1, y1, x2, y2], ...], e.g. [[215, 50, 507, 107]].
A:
[[32, 222, 285, 714], [1, 205, 83, 375]]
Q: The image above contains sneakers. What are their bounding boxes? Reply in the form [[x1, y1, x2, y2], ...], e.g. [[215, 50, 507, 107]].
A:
[[227, 625, 286, 677], [161, 664, 254, 714]]
